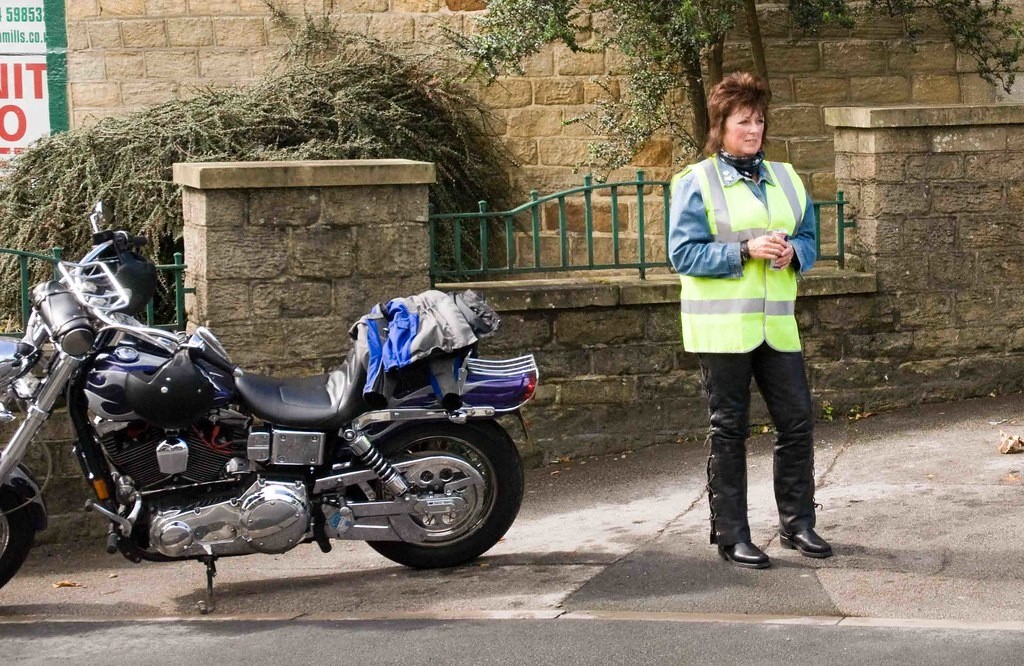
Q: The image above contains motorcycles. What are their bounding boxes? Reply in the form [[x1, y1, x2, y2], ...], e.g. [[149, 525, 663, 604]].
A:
[[0, 199, 539, 616]]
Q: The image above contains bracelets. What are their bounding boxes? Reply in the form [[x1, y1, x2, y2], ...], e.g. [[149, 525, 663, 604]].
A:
[[739, 239, 750, 262]]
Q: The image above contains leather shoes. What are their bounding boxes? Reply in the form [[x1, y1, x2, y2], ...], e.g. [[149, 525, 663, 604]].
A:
[[718, 542, 771, 568], [781, 529, 833, 557]]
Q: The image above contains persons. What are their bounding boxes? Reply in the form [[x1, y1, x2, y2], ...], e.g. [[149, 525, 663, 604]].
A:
[[668, 73, 832, 568]]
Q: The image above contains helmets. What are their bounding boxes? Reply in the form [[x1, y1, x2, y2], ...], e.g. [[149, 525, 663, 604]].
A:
[[94, 255, 157, 316]]
